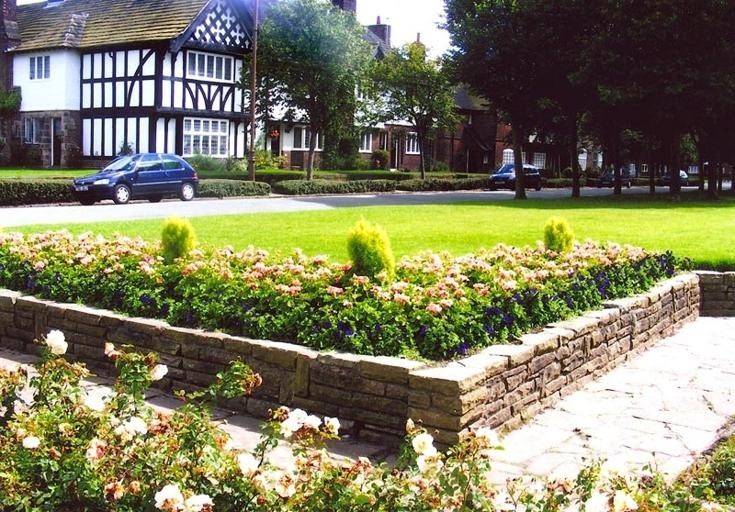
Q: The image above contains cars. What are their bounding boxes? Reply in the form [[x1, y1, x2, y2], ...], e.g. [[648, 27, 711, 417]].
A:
[[487, 162, 543, 191], [70, 153, 199, 205], [597, 167, 632, 188], [658, 169, 689, 186]]
